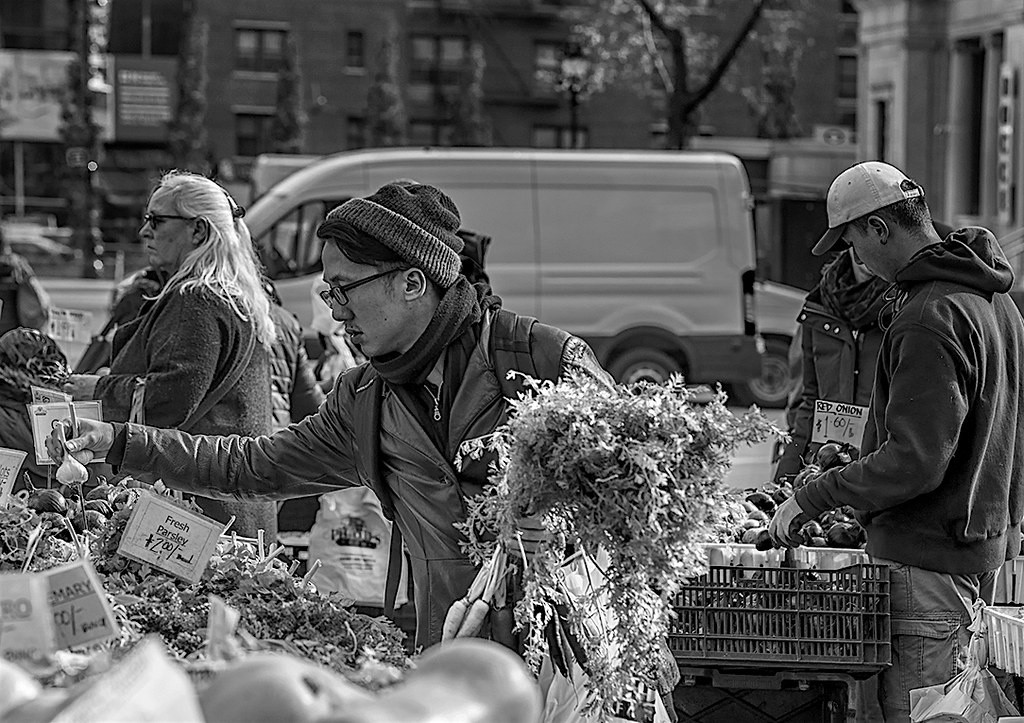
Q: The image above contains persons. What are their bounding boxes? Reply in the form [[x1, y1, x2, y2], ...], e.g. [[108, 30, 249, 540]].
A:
[[60, 172, 279, 553], [98, 242, 380, 433], [0, 226, 51, 409], [45, 181, 679, 723], [761, 161, 1024, 723]]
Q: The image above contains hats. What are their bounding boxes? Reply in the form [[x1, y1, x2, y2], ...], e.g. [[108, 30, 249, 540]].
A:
[[325, 181, 466, 289], [811, 160, 926, 256]]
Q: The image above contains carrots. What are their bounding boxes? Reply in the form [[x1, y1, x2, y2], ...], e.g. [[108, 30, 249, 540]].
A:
[[442, 599, 515, 655]]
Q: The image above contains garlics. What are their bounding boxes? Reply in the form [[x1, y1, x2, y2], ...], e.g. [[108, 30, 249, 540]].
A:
[[208, 516, 321, 592], [55, 404, 94, 487]]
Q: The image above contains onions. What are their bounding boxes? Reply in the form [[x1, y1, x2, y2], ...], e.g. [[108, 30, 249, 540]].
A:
[[740, 441, 861, 549], [23, 472, 148, 532]]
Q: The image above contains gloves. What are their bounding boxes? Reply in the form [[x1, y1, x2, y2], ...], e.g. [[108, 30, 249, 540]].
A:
[[769, 490, 805, 549]]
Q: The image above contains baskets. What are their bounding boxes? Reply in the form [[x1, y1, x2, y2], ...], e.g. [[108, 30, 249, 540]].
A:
[[666, 562, 893, 692]]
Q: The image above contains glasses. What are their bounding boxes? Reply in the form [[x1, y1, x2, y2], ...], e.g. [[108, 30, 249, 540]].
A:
[[143, 212, 196, 230], [319, 266, 409, 309]]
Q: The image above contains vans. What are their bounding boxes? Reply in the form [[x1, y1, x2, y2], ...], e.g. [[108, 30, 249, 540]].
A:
[[236, 143, 765, 404]]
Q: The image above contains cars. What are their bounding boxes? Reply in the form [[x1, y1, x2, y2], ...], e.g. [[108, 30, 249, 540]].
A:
[[709, 276, 812, 410]]
